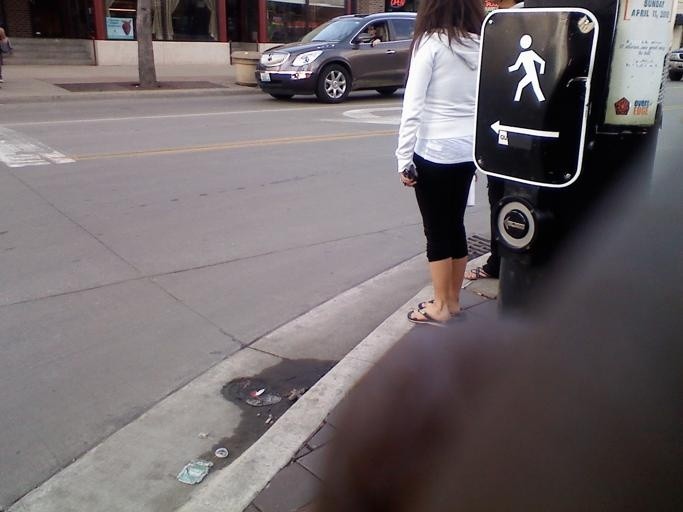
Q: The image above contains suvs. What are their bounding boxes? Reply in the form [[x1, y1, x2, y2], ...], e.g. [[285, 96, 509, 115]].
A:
[[669, 49, 683, 81], [254, 11, 418, 104]]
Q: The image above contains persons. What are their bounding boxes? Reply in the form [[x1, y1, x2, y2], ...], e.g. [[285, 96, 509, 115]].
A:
[[0, 28, 12, 84], [463, 164, 504, 282], [364, 25, 383, 47], [391, 2, 499, 329]]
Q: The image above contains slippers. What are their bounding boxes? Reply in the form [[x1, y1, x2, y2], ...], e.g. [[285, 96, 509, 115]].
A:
[[464, 267, 490, 279], [407, 299, 462, 326]]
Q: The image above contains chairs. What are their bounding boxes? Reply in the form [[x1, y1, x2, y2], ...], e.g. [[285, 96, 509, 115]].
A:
[[374, 26, 385, 42]]
